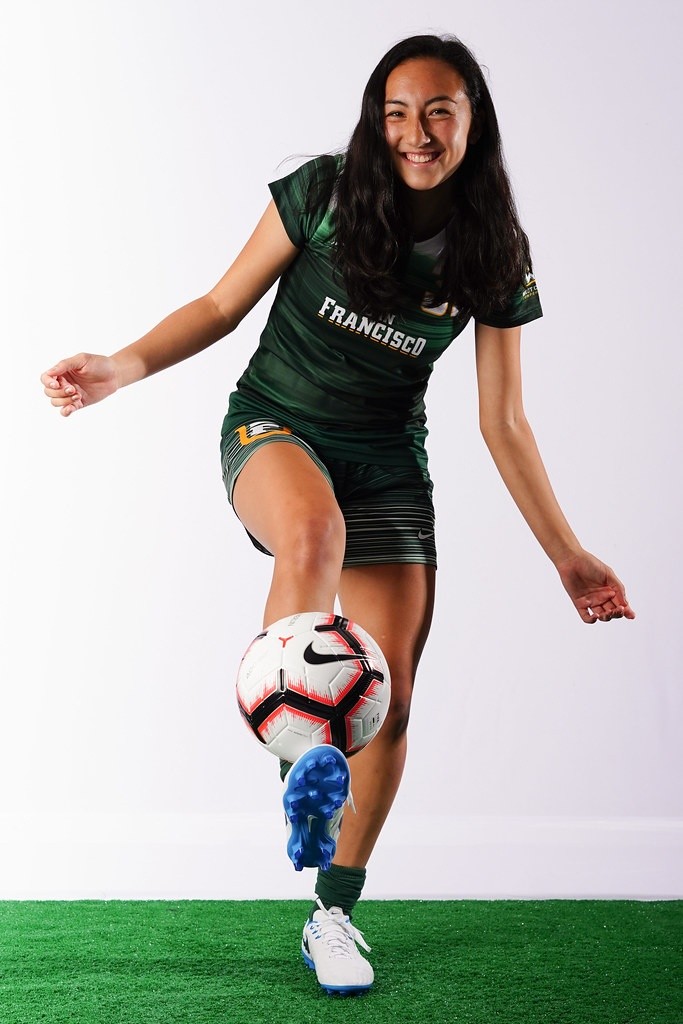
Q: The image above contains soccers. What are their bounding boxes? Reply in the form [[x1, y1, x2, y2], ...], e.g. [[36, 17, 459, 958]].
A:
[[238, 611, 393, 764]]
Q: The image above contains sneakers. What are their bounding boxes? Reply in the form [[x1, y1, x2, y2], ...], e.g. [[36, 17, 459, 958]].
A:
[[279, 744, 356, 870], [300, 898, 373, 994]]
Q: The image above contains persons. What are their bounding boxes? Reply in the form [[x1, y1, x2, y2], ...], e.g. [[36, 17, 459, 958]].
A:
[[38, 35, 636, 996]]
